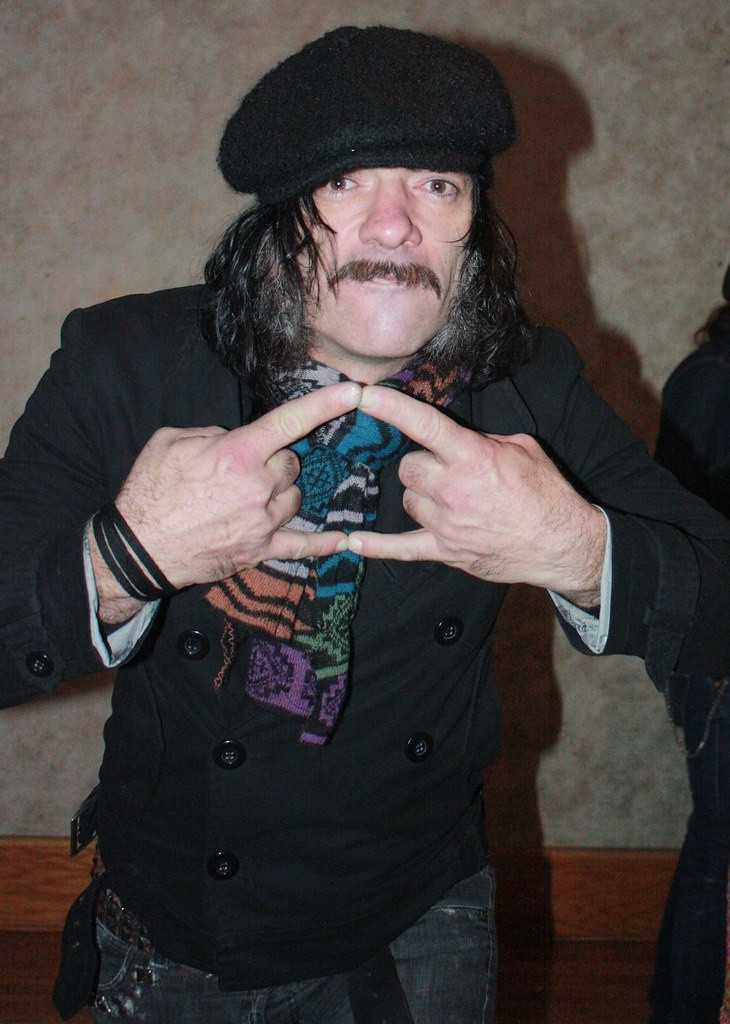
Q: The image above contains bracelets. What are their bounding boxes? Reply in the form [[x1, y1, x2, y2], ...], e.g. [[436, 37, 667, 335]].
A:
[[91, 501, 181, 604]]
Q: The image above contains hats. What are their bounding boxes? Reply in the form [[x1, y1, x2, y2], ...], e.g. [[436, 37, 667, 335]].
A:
[[217, 26, 515, 205]]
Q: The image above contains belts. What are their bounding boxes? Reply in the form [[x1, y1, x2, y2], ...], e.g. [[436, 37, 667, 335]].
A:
[[94, 887, 213, 979]]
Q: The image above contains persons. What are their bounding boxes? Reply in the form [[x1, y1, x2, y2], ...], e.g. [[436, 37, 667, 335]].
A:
[[0, 21, 730, 1024], [640, 267, 730, 1024]]
